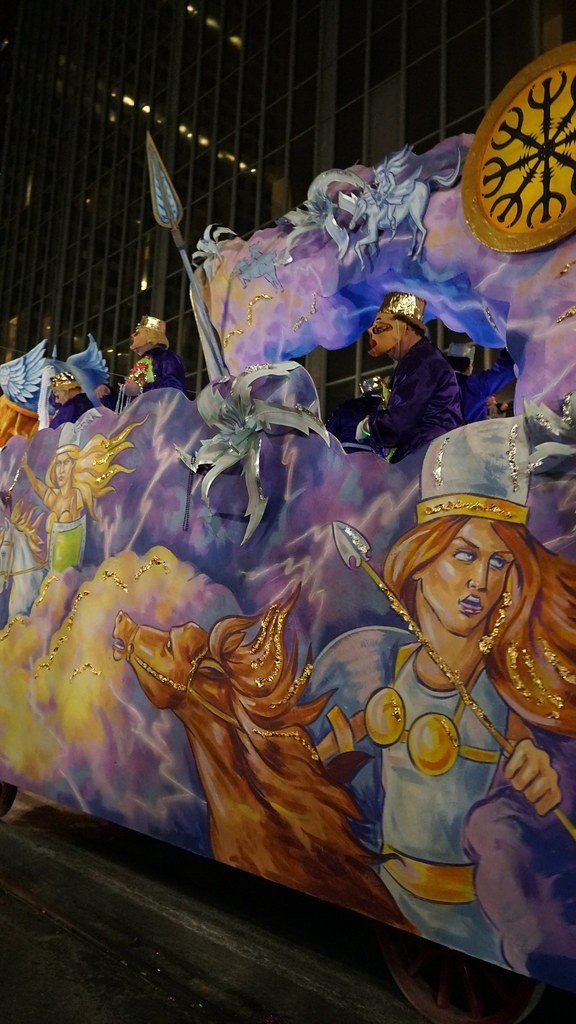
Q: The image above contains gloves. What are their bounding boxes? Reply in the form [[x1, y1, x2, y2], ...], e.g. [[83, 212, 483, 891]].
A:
[[355, 416, 371, 441]]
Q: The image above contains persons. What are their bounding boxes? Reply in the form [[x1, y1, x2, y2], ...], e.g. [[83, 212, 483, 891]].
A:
[[49, 370, 94, 429], [118, 314, 186, 397], [355, 291, 465, 463], [486, 393, 509, 420]]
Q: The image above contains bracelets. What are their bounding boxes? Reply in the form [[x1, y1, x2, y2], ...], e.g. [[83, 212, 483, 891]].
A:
[[137, 382, 144, 394], [362, 420, 371, 436]]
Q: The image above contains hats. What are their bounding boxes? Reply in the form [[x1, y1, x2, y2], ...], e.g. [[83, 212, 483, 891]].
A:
[[50, 371, 79, 386], [136, 315, 167, 334], [381, 291, 429, 332]]
